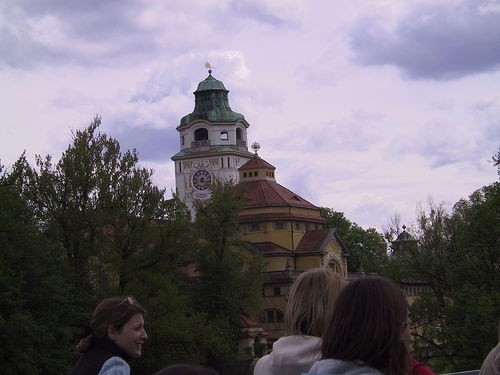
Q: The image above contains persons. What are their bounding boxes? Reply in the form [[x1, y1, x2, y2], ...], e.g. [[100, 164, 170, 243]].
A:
[[70, 296, 147, 375], [402, 310, 434, 375], [306, 274, 411, 375], [252, 267, 345, 375], [477, 343, 500, 375], [156, 364, 218, 375]]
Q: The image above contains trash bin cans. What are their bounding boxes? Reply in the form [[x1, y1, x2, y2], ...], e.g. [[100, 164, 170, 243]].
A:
[[252, 343, 267, 357]]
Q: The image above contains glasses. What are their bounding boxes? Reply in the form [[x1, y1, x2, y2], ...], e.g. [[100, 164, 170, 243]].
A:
[[108, 296, 137, 317]]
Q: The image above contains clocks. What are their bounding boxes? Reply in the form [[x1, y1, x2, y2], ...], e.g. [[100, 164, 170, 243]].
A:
[[191, 169, 213, 191]]
